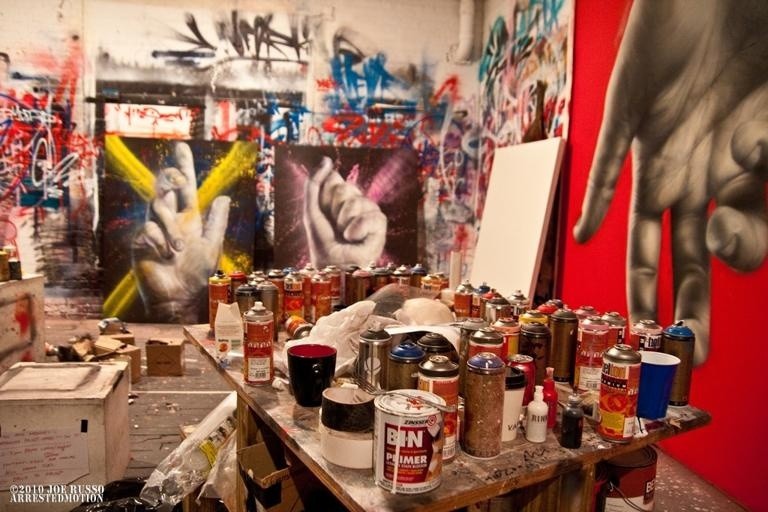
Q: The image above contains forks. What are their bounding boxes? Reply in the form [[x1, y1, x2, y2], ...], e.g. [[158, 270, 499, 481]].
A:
[[354, 374, 456, 415]]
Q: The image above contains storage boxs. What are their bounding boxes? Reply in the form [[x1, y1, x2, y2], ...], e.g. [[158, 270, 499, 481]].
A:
[[96, 317, 135, 345], [145, 336, 186, 376], [0, 360, 132, 492], [74, 339, 141, 384], [177, 423, 221, 512]]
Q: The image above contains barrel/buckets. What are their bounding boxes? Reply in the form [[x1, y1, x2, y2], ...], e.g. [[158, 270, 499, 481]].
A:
[[603, 444, 658, 512]]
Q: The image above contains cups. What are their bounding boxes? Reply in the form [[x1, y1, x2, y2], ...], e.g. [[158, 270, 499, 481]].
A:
[[501, 368, 527, 444], [638, 350, 681, 419], [320, 386, 375, 431], [286, 344, 336, 408]]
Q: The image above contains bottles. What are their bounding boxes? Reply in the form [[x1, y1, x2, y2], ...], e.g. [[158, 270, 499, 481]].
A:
[[459, 282, 475, 294], [508, 290, 531, 323], [575, 315, 616, 394], [283, 266, 297, 277], [251, 277, 264, 289], [3, 244, 16, 257], [418, 333, 451, 359], [544, 367, 557, 428], [326, 264, 342, 313], [230, 270, 247, 303], [281, 311, 314, 339], [284, 272, 302, 320], [485, 294, 510, 324], [536, 298, 559, 329], [470, 286, 490, 319], [392, 265, 410, 285], [520, 308, 549, 331], [573, 307, 597, 388], [300, 263, 317, 320], [208, 270, 231, 335], [527, 386, 549, 443], [468, 327, 505, 364], [214, 302, 244, 368], [549, 304, 579, 381], [480, 289, 501, 320], [436, 272, 449, 298], [247, 270, 266, 285], [235, 284, 259, 318], [596, 344, 642, 443], [411, 264, 427, 287], [561, 393, 583, 448], [459, 351, 504, 460], [244, 301, 273, 384], [310, 272, 333, 325], [387, 341, 425, 390], [601, 312, 625, 346], [268, 269, 285, 325], [630, 320, 664, 354], [387, 263, 398, 282], [457, 315, 487, 397], [455, 288, 471, 316], [372, 267, 391, 292], [662, 322, 694, 406], [490, 313, 520, 364], [359, 322, 392, 392], [417, 355, 459, 464], [351, 268, 372, 302], [256, 280, 278, 343], [345, 266, 360, 306], [421, 274, 439, 299], [518, 322, 551, 384]]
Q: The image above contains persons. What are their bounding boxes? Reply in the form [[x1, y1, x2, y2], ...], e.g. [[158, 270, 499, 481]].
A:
[[301, 156, 389, 272], [569, 0, 768, 368], [129, 141, 231, 322]]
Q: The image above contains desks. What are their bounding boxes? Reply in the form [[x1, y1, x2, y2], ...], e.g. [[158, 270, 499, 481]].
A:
[[182, 323, 713, 512]]
